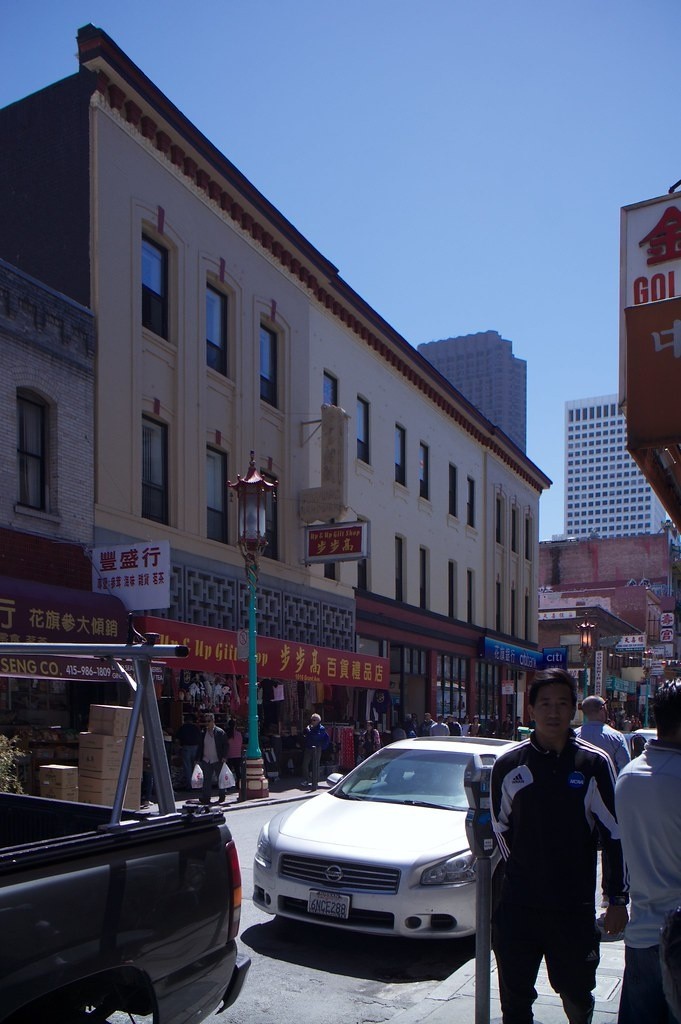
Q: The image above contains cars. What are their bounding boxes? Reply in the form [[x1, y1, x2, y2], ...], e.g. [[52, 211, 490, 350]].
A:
[[250, 735, 524, 938], [632, 728, 657, 743]]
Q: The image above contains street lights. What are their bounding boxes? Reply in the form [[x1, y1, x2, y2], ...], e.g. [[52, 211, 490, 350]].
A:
[[226, 452, 279, 798], [576, 614, 597, 727], [642, 649, 655, 728]]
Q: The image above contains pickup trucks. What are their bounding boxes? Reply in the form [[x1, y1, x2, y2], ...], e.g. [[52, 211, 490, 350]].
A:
[[1, 790, 251, 1023]]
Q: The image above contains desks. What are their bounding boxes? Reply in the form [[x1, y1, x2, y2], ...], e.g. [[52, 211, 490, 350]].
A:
[[29, 740, 79, 795]]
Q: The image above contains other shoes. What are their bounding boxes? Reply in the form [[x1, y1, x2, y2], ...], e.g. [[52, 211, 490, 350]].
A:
[[300, 781, 308, 786], [219, 797, 225, 803]]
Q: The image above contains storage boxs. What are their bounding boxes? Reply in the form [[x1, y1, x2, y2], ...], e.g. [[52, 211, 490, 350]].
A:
[[41, 787, 78, 802], [39, 764, 78, 789], [88, 704, 144, 737], [78, 776, 141, 811], [79, 731, 144, 780]]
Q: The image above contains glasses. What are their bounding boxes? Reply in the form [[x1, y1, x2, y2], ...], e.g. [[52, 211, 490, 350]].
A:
[[204, 720, 211, 723], [313, 719, 316, 722]]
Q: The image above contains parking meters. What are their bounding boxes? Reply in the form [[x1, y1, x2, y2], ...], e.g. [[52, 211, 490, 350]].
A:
[[462, 752, 503, 1024]]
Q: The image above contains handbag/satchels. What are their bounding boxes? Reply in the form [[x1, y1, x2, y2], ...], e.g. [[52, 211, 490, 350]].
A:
[[191, 764, 204, 788], [218, 762, 235, 789]]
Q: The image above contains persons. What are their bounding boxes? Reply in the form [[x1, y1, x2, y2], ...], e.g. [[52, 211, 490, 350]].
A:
[[610, 707, 655, 732], [573, 694, 632, 908], [348, 711, 536, 767], [297, 713, 327, 792], [127, 699, 155, 808], [488, 667, 629, 1024], [226, 718, 242, 792], [616, 678, 681, 1024], [177, 714, 201, 791], [193, 712, 229, 805]]
